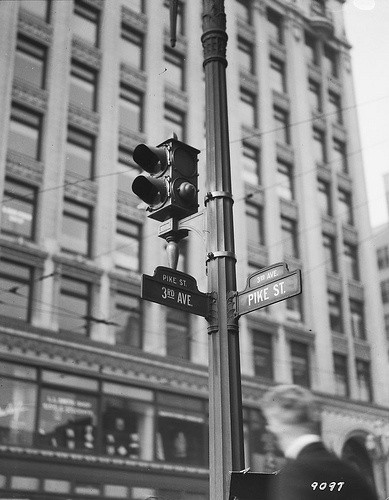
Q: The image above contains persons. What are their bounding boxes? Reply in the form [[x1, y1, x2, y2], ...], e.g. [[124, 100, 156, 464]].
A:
[[260, 384, 377, 495]]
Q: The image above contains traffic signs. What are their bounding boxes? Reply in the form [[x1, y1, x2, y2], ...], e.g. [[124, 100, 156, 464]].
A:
[[228, 262, 302, 331], [141, 265, 219, 334]]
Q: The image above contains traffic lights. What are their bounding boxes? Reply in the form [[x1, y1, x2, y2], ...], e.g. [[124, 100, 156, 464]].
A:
[[132, 138, 201, 223]]
